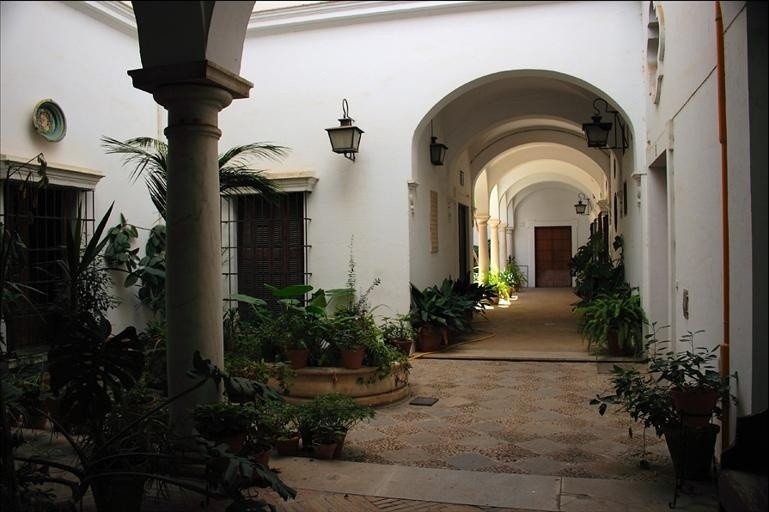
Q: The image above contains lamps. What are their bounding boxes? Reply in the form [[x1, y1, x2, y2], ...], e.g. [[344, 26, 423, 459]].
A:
[[580, 97, 631, 151], [323, 97, 363, 162], [429, 121, 448, 166], [574, 192, 588, 215]]
